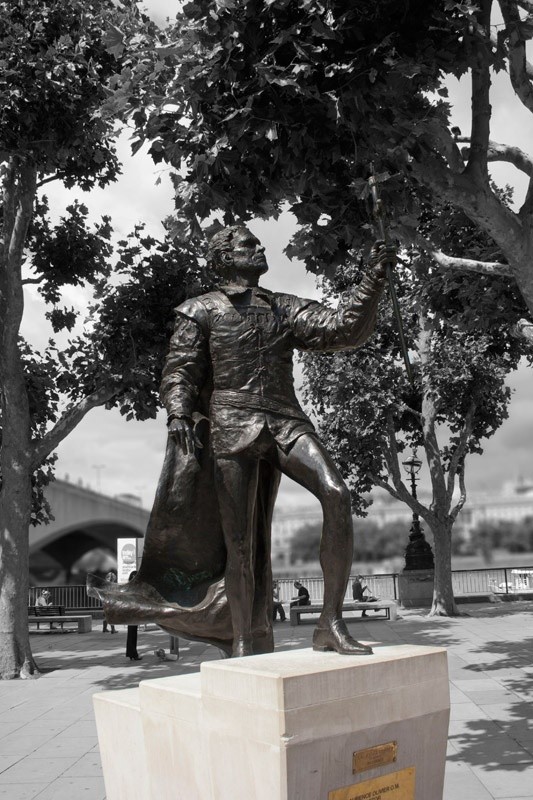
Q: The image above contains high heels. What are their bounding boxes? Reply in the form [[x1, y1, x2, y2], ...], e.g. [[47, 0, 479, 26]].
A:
[[130, 656, 143, 660]]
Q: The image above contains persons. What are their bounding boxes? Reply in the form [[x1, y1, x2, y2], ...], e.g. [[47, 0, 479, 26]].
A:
[[352, 574, 381, 617], [85, 223, 397, 658], [125, 571, 145, 662], [102, 572, 120, 634], [289, 581, 310, 608], [272, 580, 287, 622], [34, 589, 58, 630]]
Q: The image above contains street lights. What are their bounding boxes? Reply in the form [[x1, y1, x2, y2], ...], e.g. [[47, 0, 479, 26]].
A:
[[396, 448, 434, 570]]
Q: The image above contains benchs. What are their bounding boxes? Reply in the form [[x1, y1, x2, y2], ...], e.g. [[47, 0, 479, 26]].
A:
[[28, 605, 66, 630], [28, 615, 92, 634], [290, 600, 397, 626]]
